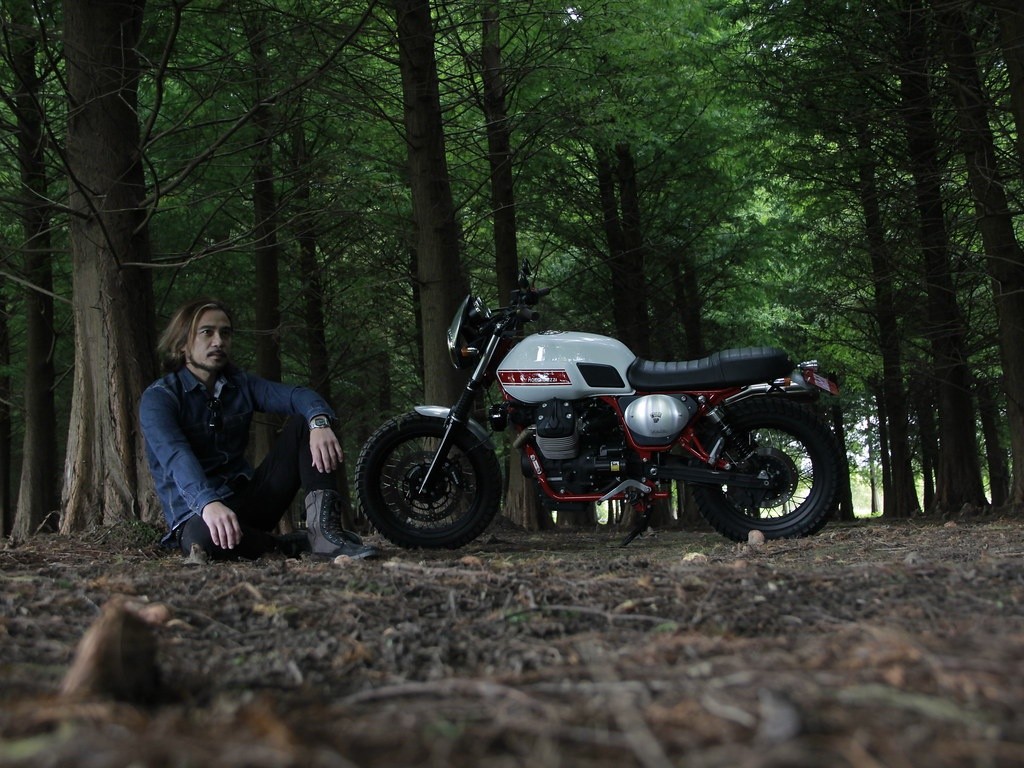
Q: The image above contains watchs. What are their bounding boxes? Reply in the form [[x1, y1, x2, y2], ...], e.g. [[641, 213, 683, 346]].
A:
[[307, 416, 330, 429]]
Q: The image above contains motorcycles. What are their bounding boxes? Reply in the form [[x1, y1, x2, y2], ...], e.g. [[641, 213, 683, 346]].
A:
[[353, 253, 850, 550]]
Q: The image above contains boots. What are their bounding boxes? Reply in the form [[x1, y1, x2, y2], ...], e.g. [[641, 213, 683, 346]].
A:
[[304, 489, 383, 560], [284, 525, 363, 559]]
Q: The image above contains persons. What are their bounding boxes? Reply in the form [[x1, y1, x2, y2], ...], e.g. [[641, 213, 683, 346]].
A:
[[136, 301, 390, 561]]
